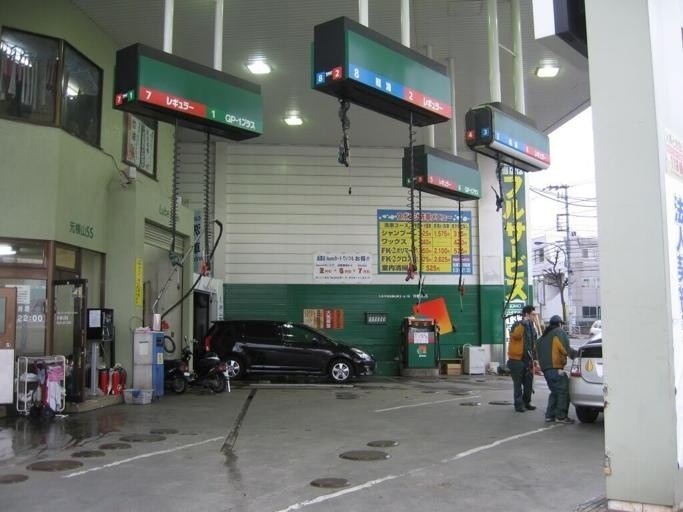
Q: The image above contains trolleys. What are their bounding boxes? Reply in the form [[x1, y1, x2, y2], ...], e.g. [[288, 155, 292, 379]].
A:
[[30, 360, 55, 419]]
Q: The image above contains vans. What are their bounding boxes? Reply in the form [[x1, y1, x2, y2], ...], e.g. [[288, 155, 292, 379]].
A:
[[202, 320, 376, 384]]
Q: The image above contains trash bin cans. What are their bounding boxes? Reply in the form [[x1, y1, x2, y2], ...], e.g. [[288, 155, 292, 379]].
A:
[[464, 346, 486, 376]]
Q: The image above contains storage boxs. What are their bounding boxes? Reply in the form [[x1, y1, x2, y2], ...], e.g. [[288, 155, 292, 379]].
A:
[[446, 363, 464, 375], [123, 388, 154, 405]]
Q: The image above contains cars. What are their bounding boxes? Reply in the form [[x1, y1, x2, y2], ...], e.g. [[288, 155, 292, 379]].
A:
[[568, 320, 603, 423]]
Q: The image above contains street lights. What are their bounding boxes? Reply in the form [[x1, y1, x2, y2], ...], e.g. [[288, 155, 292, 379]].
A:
[[535, 241, 574, 337]]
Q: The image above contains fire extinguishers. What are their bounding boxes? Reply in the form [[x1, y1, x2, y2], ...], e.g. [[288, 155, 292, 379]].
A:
[[99, 367, 126, 396]]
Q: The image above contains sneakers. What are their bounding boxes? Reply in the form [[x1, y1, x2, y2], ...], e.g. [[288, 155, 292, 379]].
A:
[[515, 404, 536, 411], [545, 415, 575, 423]]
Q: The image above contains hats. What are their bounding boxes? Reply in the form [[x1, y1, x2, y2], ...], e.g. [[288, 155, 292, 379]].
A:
[[550, 315, 565, 324]]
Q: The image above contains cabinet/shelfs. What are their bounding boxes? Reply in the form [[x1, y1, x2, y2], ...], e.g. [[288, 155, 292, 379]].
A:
[[16, 355, 66, 416]]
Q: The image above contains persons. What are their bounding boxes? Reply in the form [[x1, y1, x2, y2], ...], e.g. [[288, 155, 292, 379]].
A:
[[535, 314, 578, 425], [506, 305, 537, 413]]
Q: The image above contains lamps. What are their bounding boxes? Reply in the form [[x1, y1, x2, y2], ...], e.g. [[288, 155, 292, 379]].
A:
[[535, 66, 560, 78], [122, 166, 137, 188], [283, 116, 305, 126], [245, 62, 273, 74]]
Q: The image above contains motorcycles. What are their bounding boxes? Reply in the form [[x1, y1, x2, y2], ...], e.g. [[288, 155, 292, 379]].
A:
[[164, 361, 190, 394], [180, 337, 226, 393]]
